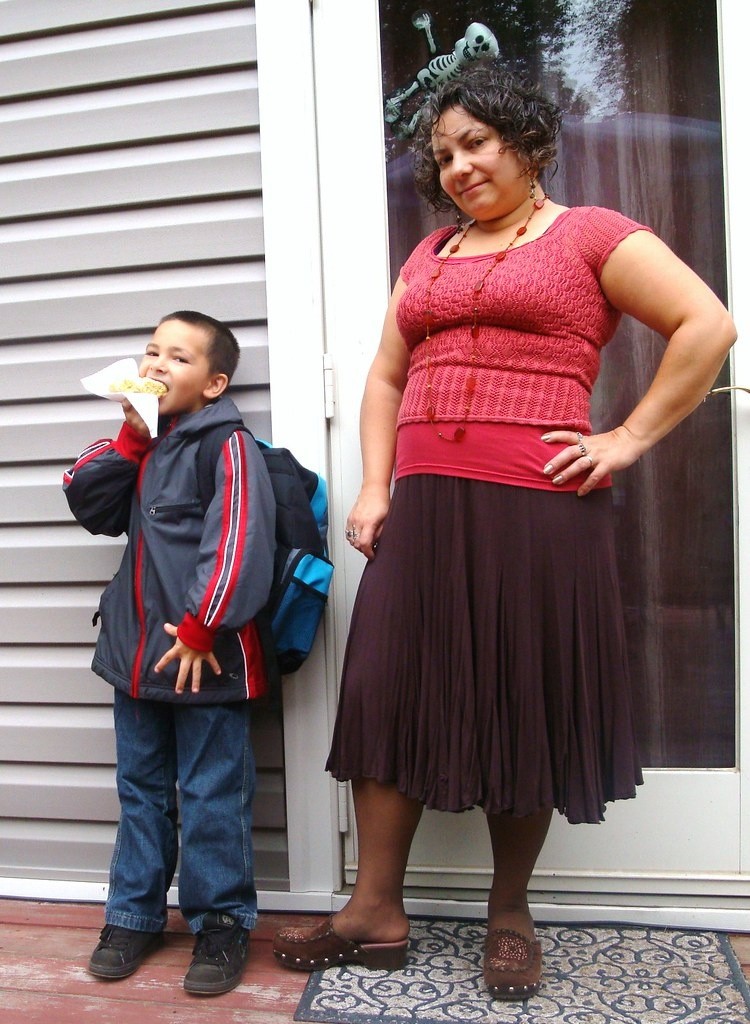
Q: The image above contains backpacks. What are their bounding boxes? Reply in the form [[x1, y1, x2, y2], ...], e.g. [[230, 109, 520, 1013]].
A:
[[198, 422, 336, 674]]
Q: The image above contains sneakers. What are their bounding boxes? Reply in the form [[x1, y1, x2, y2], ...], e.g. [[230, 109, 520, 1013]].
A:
[[184, 910, 251, 993], [89, 923, 165, 978]]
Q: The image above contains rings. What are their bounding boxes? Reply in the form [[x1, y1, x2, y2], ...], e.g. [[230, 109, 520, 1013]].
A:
[[577, 443, 587, 456], [577, 432, 583, 444], [584, 456, 594, 469], [345, 530, 358, 538]]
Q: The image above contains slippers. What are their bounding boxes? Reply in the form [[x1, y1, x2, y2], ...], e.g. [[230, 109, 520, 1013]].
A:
[[271, 914, 408, 971], [483, 928, 542, 1000]]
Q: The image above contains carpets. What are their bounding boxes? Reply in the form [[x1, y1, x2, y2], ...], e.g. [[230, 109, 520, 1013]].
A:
[[291, 917, 750, 1024]]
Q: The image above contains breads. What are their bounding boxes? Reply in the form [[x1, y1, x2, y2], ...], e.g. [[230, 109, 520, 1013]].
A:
[[107, 378, 168, 398]]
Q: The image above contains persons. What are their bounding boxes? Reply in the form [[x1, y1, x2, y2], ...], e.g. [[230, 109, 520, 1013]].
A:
[[274, 59, 737, 1000], [65, 309, 274, 996]]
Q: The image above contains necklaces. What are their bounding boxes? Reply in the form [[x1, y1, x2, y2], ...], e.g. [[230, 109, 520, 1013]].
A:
[[422, 192, 552, 444]]
